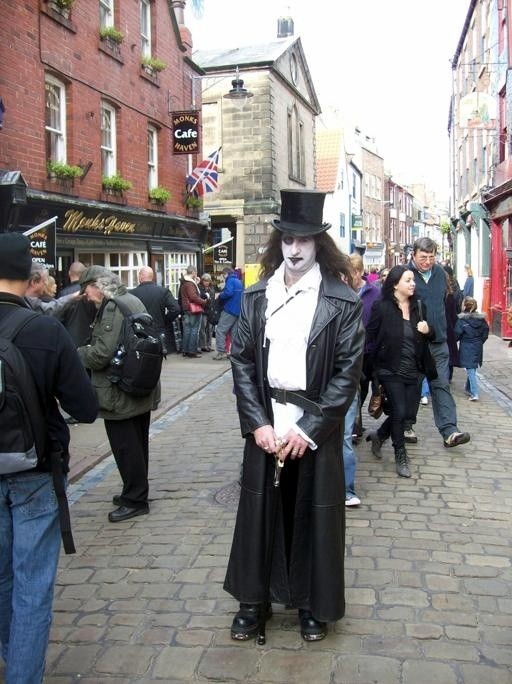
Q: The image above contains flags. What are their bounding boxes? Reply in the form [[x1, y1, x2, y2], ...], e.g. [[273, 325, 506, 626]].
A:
[[186, 151, 218, 195]]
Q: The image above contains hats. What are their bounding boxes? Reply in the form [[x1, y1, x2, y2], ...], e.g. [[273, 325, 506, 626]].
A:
[[0, 231, 33, 281], [270, 189, 332, 239], [79, 265, 109, 297]]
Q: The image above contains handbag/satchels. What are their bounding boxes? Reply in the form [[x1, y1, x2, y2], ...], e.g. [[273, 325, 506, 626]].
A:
[[190, 302, 204, 313], [207, 299, 224, 325]]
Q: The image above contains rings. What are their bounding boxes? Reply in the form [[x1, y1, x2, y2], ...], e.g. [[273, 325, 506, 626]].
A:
[[280, 439, 286, 446], [264, 447, 269, 450]]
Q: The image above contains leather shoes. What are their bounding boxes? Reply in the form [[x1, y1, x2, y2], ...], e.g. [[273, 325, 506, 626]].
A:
[[184, 348, 214, 358], [109, 496, 149, 522], [298, 608, 327, 641], [231, 605, 273, 642]]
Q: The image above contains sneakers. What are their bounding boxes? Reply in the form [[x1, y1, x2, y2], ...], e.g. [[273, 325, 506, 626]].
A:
[[444, 431, 470, 447], [213, 352, 231, 360], [366, 385, 428, 477], [464, 390, 479, 401], [345, 493, 361, 506]]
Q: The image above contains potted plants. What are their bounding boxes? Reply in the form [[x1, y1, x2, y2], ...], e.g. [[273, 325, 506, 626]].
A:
[[50, 0, 76, 19], [101, 173, 133, 197], [186, 197, 202, 211], [146, 185, 170, 206], [141, 58, 167, 78], [47, 160, 85, 189], [99, 26, 126, 54]]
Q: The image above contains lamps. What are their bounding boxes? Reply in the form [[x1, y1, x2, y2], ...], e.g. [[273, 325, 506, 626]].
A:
[[190, 65, 255, 109]]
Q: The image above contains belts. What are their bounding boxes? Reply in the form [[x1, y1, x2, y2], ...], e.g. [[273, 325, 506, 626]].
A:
[[268, 388, 323, 417]]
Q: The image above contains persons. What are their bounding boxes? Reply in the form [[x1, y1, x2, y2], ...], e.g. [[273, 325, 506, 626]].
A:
[[1, 232, 99, 683], [342, 237, 489, 506], [25, 262, 182, 521], [178, 265, 244, 360], [222, 189, 367, 642]]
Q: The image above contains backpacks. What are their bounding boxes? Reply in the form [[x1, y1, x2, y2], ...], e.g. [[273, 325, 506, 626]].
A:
[[98, 299, 163, 397], [0, 308, 50, 479]]
